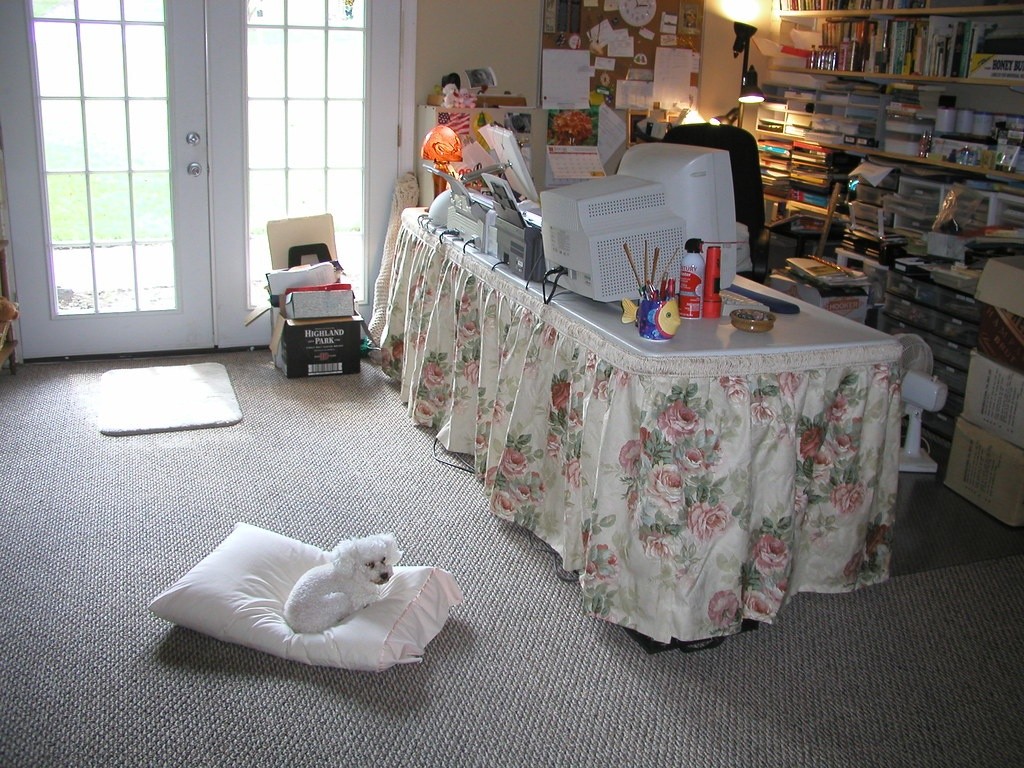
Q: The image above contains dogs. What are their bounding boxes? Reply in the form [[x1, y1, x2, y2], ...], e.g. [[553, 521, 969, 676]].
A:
[[285, 534, 404, 632]]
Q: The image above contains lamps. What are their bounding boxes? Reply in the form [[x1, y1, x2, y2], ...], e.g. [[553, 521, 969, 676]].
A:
[[707, 20, 765, 127]]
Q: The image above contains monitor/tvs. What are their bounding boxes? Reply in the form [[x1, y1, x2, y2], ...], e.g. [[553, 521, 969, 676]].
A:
[[542, 142, 737, 303]]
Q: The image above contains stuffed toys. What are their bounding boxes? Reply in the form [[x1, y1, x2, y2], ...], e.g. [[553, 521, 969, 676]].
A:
[[442, 83, 477, 108]]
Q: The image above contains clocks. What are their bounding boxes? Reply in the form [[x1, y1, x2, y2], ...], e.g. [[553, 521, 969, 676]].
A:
[[618, 0, 657, 27]]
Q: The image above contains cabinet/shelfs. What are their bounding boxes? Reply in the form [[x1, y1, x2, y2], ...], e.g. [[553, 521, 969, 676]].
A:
[[751, 0, 1024, 226], [0, 239, 19, 376]]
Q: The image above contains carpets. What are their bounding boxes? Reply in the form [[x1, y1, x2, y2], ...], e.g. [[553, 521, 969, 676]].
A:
[[889, 473, 1024, 588], [99, 359, 242, 435]]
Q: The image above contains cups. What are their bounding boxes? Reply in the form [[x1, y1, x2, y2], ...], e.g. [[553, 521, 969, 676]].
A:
[[622, 296, 681, 343]]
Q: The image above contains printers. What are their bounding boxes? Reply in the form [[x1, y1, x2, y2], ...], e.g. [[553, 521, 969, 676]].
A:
[[427, 167, 549, 282]]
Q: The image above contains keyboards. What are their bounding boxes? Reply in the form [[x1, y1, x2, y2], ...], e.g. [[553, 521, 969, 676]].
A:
[[718, 290, 772, 316]]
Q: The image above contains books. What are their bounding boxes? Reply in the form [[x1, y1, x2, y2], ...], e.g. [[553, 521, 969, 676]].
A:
[[780, 1, 928, 11], [754, 137, 863, 213], [822, 21, 985, 77]]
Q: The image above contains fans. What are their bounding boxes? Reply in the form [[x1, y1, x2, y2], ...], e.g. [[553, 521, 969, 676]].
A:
[[894, 333, 949, 475]]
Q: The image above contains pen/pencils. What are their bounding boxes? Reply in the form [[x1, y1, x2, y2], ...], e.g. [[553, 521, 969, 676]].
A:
[[639, 272, 676, 301]]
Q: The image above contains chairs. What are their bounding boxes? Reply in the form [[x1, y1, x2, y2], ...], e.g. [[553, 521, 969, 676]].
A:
[[664, 121, 772, 284]]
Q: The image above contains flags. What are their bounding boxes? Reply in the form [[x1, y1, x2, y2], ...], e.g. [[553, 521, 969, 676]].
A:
[[437, 112, 470, 134]]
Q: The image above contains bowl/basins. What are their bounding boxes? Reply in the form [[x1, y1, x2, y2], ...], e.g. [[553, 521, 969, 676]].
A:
[[730, 308, 776, 333]]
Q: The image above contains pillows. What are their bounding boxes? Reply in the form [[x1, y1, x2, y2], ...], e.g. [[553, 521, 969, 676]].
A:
[[145, 521, 463, 676]]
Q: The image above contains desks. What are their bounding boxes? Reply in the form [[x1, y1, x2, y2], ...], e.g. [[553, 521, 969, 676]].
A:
[[373, 204, 907, 655]]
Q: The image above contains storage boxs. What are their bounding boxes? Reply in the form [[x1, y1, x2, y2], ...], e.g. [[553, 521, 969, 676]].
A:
[[848, 201, 892, 239], [966, 53, 1024, 82], [758, 81, 997, 167], [284, 290, 356, 318], [894, 176, 944, 236], [974, 254, 1024, 374], [266, 260, 343, 309], [959, 349, 1024, 451], [269, 310, 380, 380], [770, 265, 868, 327], [942, 172, 1002, 239], [942, 416, 1024, 527]]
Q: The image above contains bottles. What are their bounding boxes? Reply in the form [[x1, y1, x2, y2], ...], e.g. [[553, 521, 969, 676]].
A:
[[803, 37, 852, 71], [679, 238, 705, 319], [918, 129, 933, 158]]
